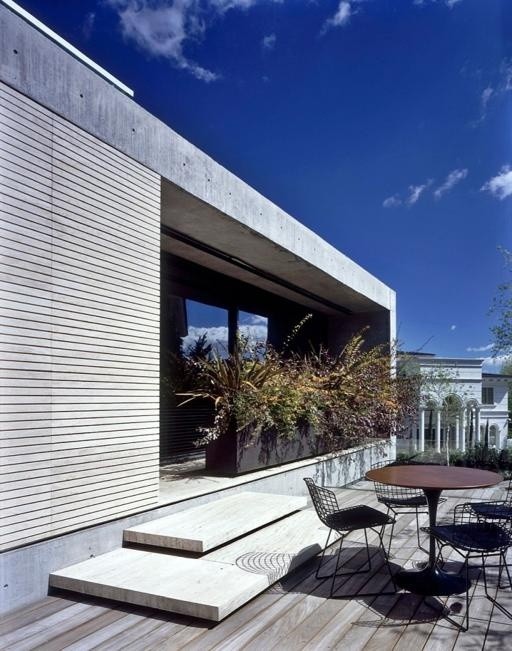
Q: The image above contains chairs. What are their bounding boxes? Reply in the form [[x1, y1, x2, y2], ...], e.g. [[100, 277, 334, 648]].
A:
[[370, 460, 447, 563], [419, 498, 512, 631], [304, 478, 396, 597], [454, 473, 511, 535]]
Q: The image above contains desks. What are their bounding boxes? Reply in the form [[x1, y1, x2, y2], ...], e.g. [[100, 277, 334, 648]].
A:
[[365, 465, 504, 596]]
